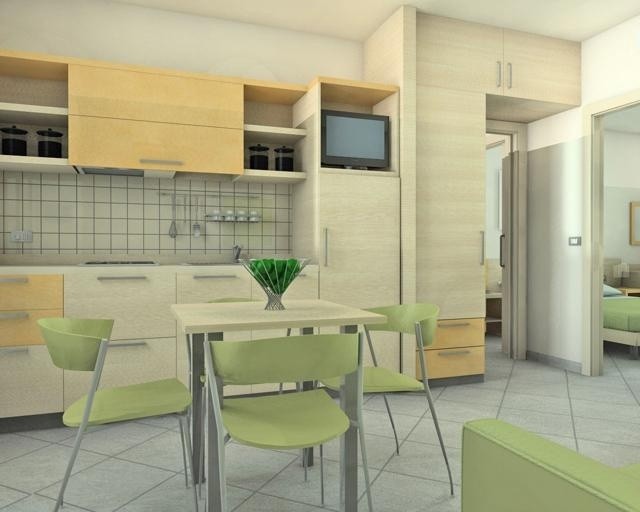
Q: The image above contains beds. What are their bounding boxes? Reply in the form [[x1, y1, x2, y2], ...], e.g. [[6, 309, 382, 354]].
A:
[[603, 294, 640, 360]]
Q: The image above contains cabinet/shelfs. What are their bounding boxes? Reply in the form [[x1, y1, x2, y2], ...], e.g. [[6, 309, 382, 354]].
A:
[[0, 50, 309, 180], [64, 266, 176, 411], [485, 292, 503, 337], [251, 265, 319, 394], [1, 267, 64, 433], [290, 78, 401, 382], [416, 10, 583, 383], [177, 266, 253, 400]]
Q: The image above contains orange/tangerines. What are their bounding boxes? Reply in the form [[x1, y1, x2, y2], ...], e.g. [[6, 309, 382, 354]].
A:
[[249, 259, 301, 295]]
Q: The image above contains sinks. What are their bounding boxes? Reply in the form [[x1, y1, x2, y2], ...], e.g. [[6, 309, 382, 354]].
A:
[[186, 261, 244, 266]]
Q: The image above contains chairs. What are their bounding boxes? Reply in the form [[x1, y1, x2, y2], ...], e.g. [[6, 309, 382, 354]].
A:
[[462, 417, 640, 512]]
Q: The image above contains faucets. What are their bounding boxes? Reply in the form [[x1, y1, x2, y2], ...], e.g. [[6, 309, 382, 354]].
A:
[[232, 245, 243, 261]]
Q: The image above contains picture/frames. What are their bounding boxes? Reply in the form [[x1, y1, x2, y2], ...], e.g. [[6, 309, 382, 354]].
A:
[[630, 201, 640, 246]]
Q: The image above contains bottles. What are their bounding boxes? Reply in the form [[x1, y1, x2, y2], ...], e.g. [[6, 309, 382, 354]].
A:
[[212, 209, 221, 220], [224, 210, 235, 221], [249, 210, 261, 221], [237, 210, 248, 222], [0, 125, 28, 156], [248, 144, 269, 170], [36, 128, 64, 157], [274, 145, 295, 172]]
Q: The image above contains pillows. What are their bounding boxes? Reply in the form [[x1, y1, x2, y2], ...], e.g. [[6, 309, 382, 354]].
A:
[[603, 284, 622, 297]]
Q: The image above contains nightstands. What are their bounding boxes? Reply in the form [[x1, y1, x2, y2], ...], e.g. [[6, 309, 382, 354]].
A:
[[618, 287, 640, 296]]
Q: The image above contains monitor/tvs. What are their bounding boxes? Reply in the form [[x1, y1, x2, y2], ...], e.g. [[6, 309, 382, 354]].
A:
[[321, 109, 389, 170]]
